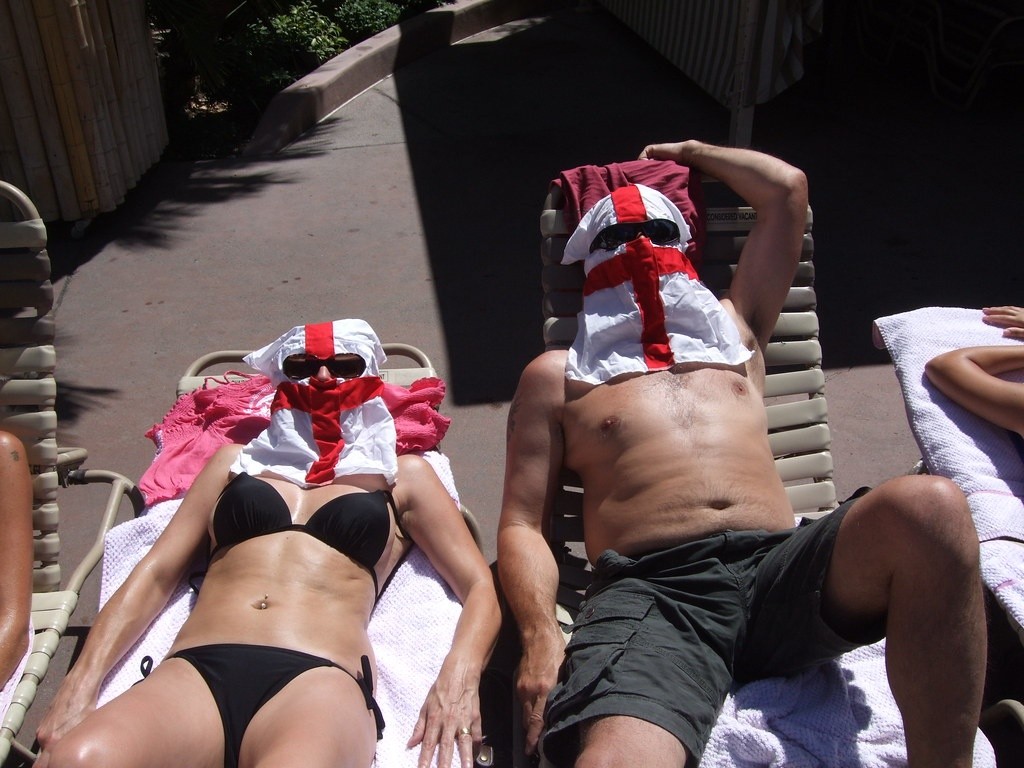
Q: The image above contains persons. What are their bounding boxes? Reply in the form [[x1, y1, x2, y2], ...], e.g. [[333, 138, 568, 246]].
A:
[[925, 305, 1024, 438], [497, 137, 989, 768], [0, 430, 33, 691], [34, 316, 503, 768]]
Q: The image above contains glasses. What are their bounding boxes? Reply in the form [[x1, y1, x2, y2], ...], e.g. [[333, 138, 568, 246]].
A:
[[283, 358, 366, 380], [589, 219, 680, 255]]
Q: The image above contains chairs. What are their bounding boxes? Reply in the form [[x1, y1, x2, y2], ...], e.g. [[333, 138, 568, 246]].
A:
[[0, 177, 143, 768], [96, 340, 481, 768], [872, 306, 1024, 735], [538, 188, 997, 768]]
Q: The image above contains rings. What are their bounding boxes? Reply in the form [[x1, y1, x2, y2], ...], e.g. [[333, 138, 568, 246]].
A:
[[458, 728, 472, 736]]
